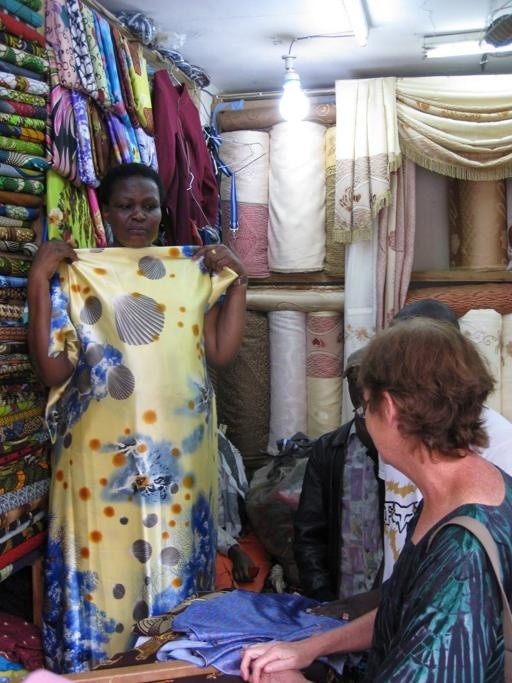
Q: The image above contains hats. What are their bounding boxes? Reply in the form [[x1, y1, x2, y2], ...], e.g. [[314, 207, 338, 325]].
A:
[[342, 348, 364, 379]]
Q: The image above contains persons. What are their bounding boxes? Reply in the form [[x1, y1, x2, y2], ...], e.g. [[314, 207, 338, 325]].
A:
[[293, 345, 424, 602], [216, 429, 255, 583], [390, 298, 511, 477], [26, 162, 249, 673], [239, 318, 511, 683]]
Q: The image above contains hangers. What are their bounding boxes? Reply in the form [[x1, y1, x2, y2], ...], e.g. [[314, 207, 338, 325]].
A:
[[167, 54, 264, 242]]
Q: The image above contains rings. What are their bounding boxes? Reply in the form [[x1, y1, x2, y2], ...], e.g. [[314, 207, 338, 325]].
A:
[[211, 249, 216, 255]]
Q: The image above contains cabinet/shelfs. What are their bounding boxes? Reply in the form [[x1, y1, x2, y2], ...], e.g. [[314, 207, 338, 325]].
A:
[[210, 94, 512, 285]]
